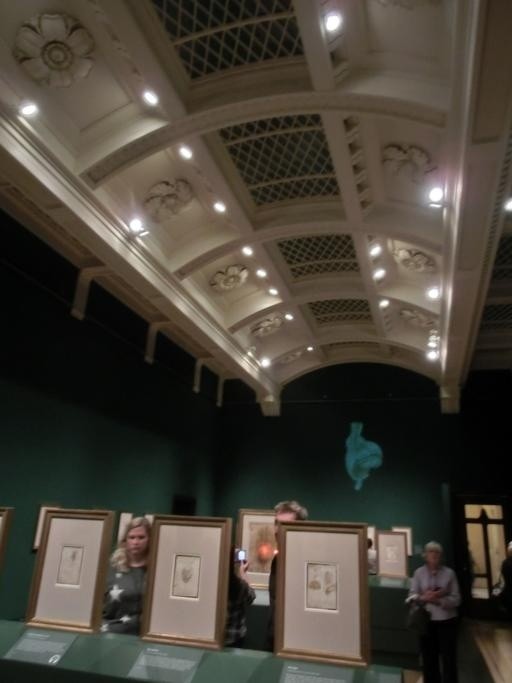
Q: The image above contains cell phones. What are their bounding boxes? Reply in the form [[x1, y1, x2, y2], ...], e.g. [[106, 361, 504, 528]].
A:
[[238, 550, 246, 561]]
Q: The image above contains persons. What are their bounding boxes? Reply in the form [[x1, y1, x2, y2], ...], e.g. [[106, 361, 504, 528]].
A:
[[405, 540, 461, 683], [224, 545, 256, 647], [490, 540, 512, 618], [102, 517, 152, 634], [265, 501, 307, 651], [367, 538, 376, 575]]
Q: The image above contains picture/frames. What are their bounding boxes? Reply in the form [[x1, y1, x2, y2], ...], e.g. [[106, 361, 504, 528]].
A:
[[140, 515, 233, 651], [235, 509, 277, 590], [367, 525, 412, 578], [273, 520, 371, 668], [25, 504, 116, 635]]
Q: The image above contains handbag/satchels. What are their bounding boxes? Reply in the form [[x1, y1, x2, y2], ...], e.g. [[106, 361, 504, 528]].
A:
[[407, 605, 431, 634]]
